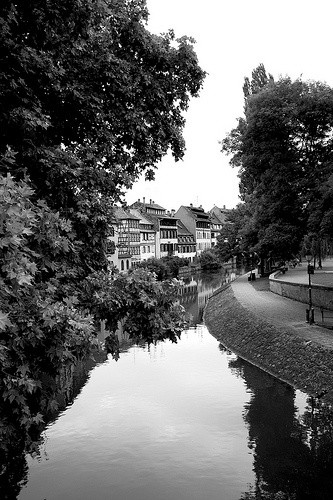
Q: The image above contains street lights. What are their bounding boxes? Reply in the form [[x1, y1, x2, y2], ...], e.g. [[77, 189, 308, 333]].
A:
[[305, 249, 314, 325]]
